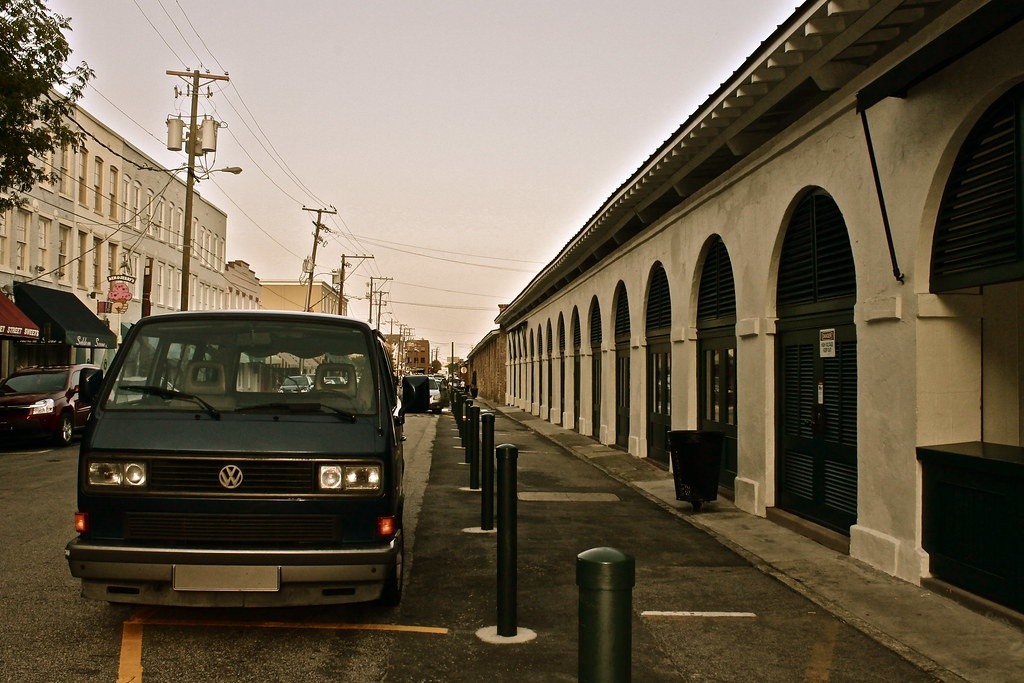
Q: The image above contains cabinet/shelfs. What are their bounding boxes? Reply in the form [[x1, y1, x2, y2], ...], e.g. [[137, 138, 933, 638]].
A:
[[915, 439, 1024, 615]]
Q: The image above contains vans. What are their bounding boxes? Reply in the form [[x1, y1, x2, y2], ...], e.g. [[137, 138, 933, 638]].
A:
[[65, 309, 430, 623]]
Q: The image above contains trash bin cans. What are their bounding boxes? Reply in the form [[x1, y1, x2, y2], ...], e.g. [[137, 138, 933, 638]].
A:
[[667, 428, 724, 510]]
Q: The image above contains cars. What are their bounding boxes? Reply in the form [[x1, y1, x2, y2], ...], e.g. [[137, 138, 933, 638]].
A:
[[413, 373, 450, 414]]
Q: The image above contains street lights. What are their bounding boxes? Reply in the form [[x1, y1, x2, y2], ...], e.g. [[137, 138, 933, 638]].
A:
[[339, 261, 352, 315], [181, 165, 243, 312], [308, 235, 328, 312]]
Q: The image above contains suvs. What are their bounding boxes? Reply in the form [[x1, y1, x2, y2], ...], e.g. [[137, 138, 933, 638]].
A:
[[1, 364, 104, 447]]
